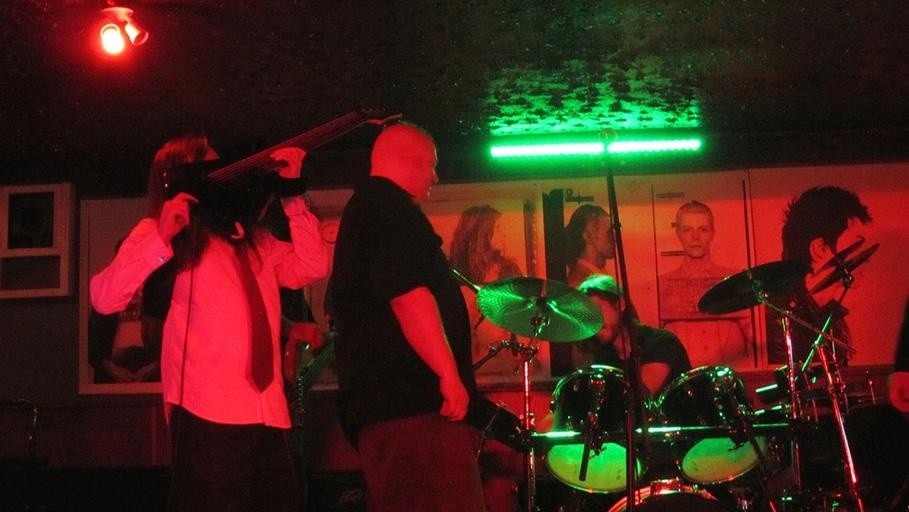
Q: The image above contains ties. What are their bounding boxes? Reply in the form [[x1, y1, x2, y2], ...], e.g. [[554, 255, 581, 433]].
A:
[[236, 247, 274, 393]]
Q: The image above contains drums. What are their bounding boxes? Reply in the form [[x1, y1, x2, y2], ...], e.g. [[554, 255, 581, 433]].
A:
[[657, 363, 769, 485], [607, 475, 735, 512], [703, 404, 849, 511], [541, 364, 654, 495]]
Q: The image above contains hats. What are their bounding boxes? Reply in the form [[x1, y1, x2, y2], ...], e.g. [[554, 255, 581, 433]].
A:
[[578, 274, 618, 296]]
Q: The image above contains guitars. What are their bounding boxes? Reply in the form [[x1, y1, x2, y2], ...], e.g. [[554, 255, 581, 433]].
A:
[[281, 322, 336, 390], [170, 102, 402, 238]]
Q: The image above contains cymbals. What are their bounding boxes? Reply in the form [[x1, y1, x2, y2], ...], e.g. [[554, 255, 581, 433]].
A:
[[475, 277, 604, 343], [809, 239, 865, 280], [811, 244, 879, 290], [697, 261, 805, 314]]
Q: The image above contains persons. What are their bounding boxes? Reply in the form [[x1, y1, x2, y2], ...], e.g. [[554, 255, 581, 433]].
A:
[[765, 181, 877, 364], [565, 271, 692, 398], [660, 201, 755, 370], [323, 120, 491, 512], [886, 296, 909, 414], [87, 241, 164, 383], [88, 133, 329, 511], [566, 204, 618, 291], [448, 205, 525, 376]]
